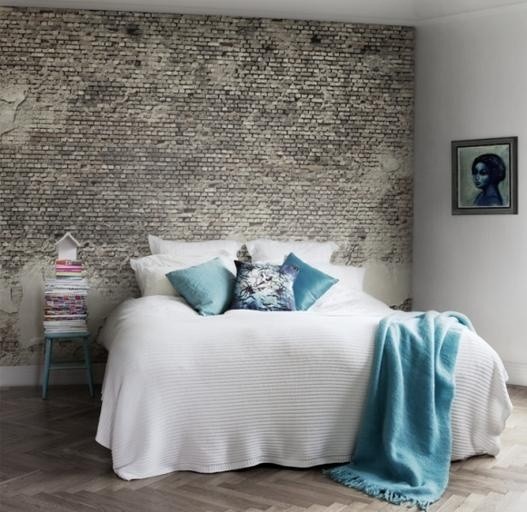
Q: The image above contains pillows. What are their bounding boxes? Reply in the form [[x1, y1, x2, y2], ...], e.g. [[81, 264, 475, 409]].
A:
[[128, 233, 342, 316]]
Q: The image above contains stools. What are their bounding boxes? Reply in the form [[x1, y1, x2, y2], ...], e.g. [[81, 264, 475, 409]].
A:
[[40, 331, 94, 400]]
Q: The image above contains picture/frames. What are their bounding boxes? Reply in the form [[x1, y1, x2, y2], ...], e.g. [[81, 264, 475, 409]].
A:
[[450, 136, 518, 216]]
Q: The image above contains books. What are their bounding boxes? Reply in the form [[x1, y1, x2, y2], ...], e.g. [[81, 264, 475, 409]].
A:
[[41, 259, 90, 333]]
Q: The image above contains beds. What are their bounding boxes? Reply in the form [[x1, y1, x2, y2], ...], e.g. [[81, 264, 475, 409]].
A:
[[93, 294, 513, 482]]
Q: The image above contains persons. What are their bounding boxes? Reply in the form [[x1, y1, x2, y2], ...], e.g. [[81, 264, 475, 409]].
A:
[[472, 153, 506, 207]]
